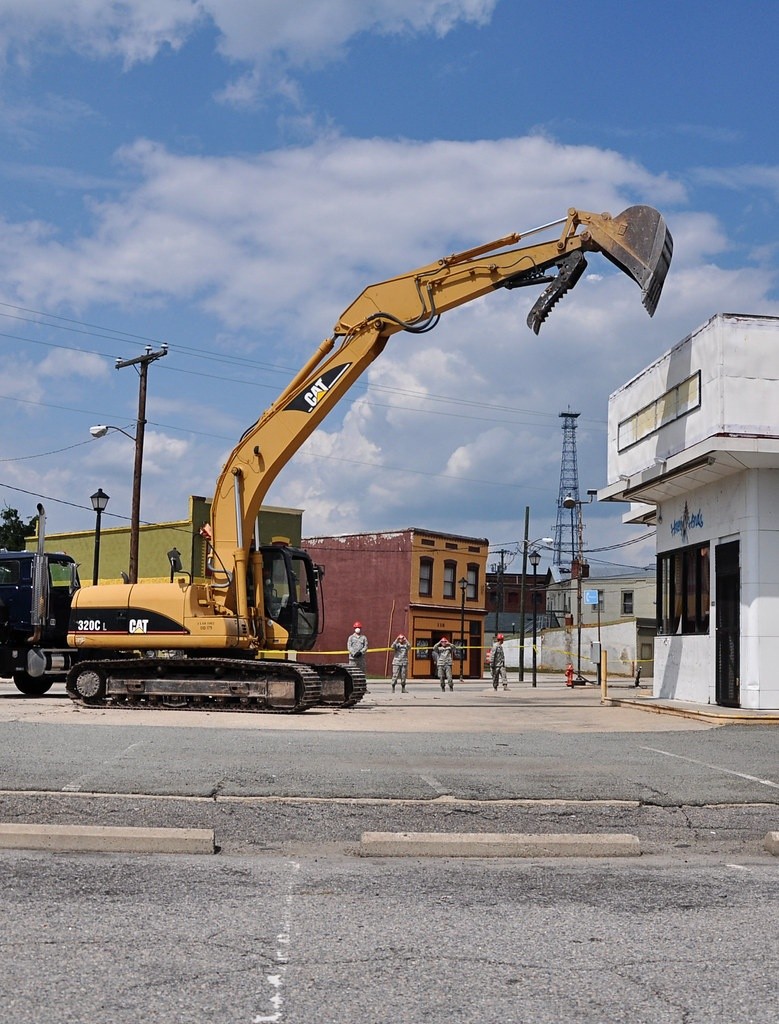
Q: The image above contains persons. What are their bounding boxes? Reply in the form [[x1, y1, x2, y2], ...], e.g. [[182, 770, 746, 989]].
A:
[[262, 569, 285, 618], [490, 634, 511, 691], [390, 634, 410, 694], [433, 638, 457, 692], [347, 622, 370, 695]]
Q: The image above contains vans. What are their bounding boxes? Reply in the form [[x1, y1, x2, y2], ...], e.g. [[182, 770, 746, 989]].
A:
[[0, 504, 82, 696]]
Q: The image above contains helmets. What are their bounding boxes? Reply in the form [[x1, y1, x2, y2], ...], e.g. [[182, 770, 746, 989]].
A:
[[354, 622, 362, 627], [497, 633, 503, 639], [440, 638, 447, 642], [398, 634, 405, 638]]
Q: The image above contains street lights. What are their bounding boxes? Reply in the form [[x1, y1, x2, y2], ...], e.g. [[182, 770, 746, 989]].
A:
[[458, 576, 468, 680], [518, 537, 553, 679], [90, 425, 144, 583], [528, 551, 541, 688], [90, 489, 110, 583]]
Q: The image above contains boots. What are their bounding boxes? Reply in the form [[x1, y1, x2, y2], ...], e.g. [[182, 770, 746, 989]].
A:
[[401, 684, 409, 693], [391, 685, 395, 693]]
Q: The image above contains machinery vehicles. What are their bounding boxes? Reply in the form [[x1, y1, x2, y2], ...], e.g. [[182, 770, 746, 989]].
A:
[[66, 204, 675, 714]]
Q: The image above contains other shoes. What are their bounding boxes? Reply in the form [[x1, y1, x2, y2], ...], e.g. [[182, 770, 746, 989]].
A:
[[493, 688, 497, 691], [450, 686, 453, 691], [504, 685, 512, 691], [365, 691, 370, 694], [442, 687, 445, 692]]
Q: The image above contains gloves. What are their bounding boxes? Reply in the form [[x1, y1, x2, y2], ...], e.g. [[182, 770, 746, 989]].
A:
[[354, 652, 362, 658]]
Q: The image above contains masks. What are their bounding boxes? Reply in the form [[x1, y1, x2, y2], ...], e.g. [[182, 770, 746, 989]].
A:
[[355, 628, 360, 634]]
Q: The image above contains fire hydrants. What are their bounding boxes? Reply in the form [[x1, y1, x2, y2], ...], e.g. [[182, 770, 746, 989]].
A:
[[564, 663, 573, 687]]
